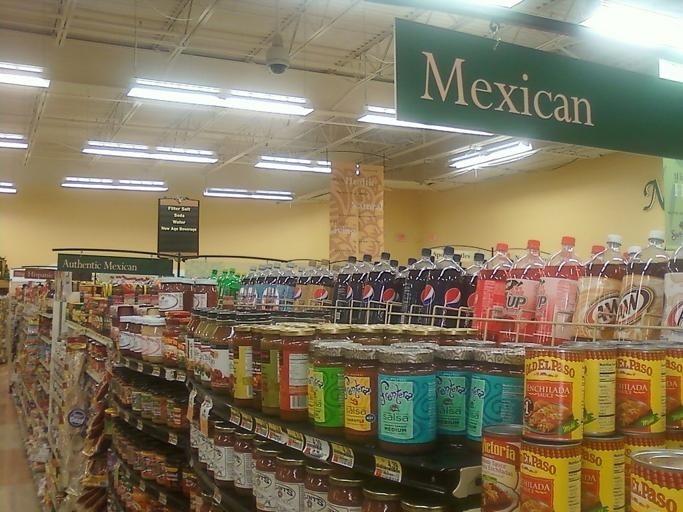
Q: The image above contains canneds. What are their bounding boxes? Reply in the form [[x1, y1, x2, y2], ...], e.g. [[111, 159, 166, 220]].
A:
[[112, 277, 683, 512]]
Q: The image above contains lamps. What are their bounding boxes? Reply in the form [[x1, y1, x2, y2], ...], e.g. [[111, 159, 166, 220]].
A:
[[0, 0, 540, 203]]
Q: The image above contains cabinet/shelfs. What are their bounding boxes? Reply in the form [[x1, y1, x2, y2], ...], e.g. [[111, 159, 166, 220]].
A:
[[0, 269, 683, 512]]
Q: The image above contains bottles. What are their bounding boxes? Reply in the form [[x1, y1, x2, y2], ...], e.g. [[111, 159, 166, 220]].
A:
[[105, 274, 158, 305], [206, 228, 682, 346]]
